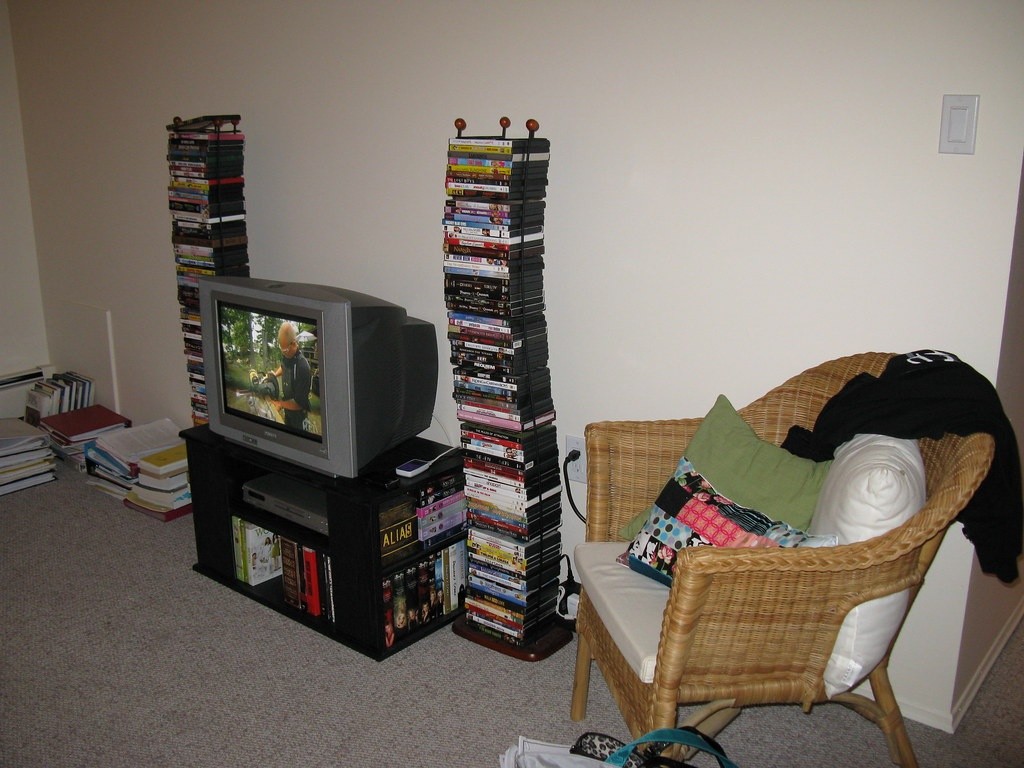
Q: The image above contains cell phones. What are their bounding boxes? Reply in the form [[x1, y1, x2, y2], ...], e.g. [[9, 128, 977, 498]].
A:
[[357, 471, 400, 488]]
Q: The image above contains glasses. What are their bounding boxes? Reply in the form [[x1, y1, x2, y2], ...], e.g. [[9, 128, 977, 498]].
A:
[[278, 344, 291, 353]]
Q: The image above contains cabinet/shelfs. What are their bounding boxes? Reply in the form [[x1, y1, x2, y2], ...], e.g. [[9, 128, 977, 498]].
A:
[[178, 422, 468, 663]]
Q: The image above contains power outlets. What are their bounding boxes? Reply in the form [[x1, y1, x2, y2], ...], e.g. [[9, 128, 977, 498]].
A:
[[566, 434, 587, 484]]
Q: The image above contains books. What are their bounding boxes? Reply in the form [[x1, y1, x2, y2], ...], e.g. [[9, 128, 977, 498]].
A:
[[231, 515, 282, 587], [301, 546, 321, 618], [0, 417, 59, 496], [123, 442, 192, 523], [321, 554, 336, 626], [38, 404, 132, 474], [280, 536, 307, 612], [24, 371, 95, 429], [83, 418, 186, 502]]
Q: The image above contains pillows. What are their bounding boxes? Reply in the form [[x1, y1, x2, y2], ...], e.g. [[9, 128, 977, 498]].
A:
[[616, 457, 838, 587], [804, 434, 926, 700], [615, 394, 834, 588]]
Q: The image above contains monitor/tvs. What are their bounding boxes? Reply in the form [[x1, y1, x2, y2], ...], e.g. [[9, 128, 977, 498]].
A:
[[198, 275, 438, 478]]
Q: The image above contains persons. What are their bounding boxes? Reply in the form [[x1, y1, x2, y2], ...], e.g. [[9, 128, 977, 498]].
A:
[[263, 321, 312, 431]]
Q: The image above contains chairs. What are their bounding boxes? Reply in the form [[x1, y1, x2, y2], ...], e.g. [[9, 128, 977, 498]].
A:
[[571, 351, 996, 768]]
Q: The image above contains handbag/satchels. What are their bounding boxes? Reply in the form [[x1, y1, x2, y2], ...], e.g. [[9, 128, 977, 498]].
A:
[[501, 725, 738, 768]]
